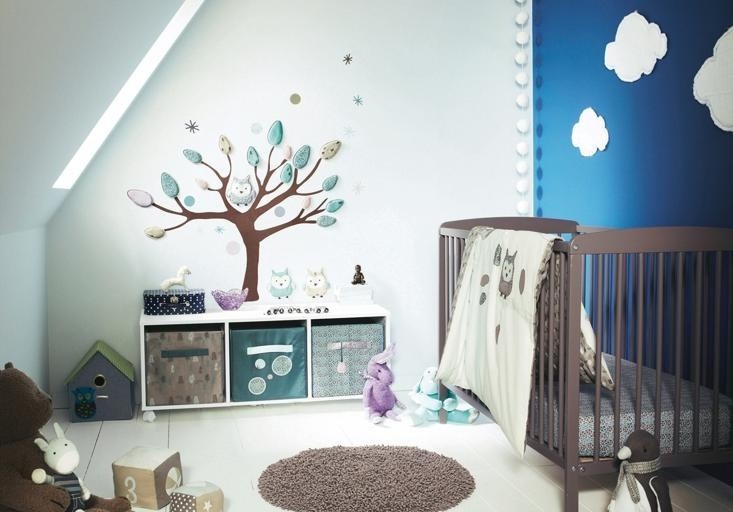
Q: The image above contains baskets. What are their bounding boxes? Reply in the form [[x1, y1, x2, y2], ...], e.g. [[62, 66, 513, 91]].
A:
[[212, 288, 249, 310]]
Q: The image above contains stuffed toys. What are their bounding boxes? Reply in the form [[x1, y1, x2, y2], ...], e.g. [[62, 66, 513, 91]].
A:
[[32, 421, 91, 512], [406, 367, 480, 426], [607, 429, 673, 511], [0, 361, 132, 512], [363, 342, 405, 427]]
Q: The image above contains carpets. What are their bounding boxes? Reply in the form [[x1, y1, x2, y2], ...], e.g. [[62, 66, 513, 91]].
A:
[[258, 444, 476, 512]]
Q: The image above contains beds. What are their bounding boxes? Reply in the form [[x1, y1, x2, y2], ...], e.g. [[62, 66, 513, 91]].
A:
[[437, 218, 732, 511]]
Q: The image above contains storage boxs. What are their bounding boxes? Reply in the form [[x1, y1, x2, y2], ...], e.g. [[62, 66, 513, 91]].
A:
[[310, 323, 383, 398], [228, 327, 307, 402], [143, 289, 205, 315], [146, 331, 225, 406]]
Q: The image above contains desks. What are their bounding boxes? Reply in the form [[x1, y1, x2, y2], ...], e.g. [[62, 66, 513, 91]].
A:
[[139, 298, 391, 422]]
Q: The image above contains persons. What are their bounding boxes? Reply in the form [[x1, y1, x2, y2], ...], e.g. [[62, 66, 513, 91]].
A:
[[352, 264, 366, 285]]
